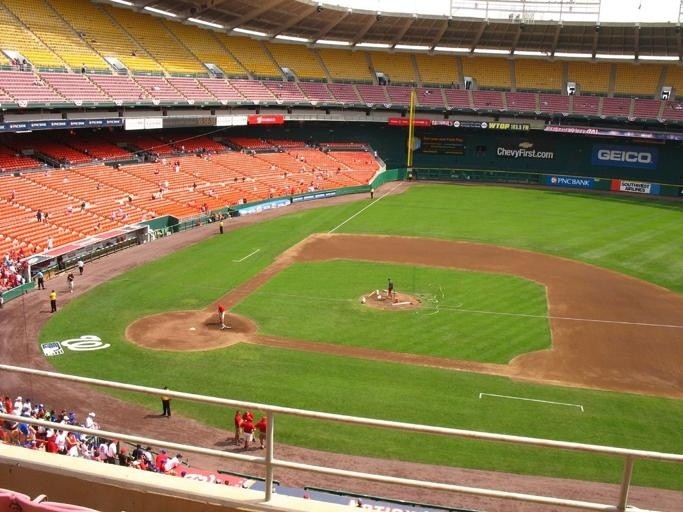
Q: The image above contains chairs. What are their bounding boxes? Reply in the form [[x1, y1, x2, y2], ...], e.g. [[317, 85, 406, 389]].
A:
[[0, 489, 89, 512]]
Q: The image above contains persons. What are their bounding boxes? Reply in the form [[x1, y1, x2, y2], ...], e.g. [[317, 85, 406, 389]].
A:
[[0, 135, 379, 307], [1, 386, 364, 510], [81, 63, 88, 74], [386, 278, 393, 300], [217, 301, 225, 329], [12, 58, 27, 72], [49, 290, 56, 313]]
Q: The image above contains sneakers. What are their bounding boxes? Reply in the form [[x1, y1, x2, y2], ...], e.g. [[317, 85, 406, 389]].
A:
[[236, 439, 265, 450]]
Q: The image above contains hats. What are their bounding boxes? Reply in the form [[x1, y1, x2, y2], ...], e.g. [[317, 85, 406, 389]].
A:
[[242, 411, 267, 422]]
